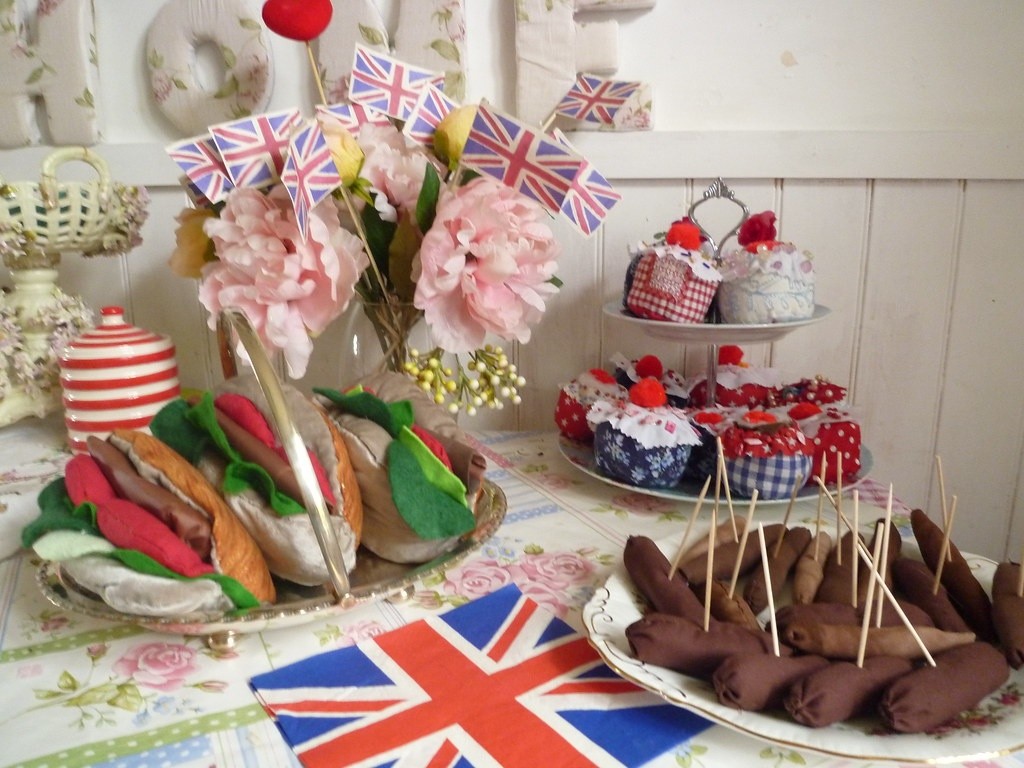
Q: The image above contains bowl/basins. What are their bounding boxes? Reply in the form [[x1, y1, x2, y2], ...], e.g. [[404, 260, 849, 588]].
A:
[[36, 476, 507, 651]]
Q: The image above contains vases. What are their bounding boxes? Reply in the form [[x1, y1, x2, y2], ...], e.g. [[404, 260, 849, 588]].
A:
[[337, 301, 464, 421]]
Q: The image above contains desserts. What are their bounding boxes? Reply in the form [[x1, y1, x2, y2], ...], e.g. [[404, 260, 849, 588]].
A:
[[554, 344, 863, 501], [622, 209, 814, 326]]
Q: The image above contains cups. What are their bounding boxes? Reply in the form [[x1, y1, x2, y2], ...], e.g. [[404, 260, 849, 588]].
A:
[[59, 306, 181, 457]]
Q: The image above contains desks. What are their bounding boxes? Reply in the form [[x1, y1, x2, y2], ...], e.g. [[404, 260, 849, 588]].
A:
[[0, 414, 1024, 768]]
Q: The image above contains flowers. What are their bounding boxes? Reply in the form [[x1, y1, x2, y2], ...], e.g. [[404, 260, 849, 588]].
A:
[[169, 121, 564, 419]]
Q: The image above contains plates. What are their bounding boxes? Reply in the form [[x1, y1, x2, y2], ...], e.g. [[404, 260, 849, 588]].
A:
[[580, 519, 1024, 764]]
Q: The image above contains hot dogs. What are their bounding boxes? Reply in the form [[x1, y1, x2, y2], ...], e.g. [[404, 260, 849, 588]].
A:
[[18, 371, 492, 618]]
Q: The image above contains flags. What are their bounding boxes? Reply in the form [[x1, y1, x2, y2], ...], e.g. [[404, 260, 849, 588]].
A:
[[280, 123, 341, 246], [457, 99, 583, 214], [168, 136, 235, 204], [316, 101, 398, 142], [177, 174, 215, 209], [402, 82, 462, 148], [525, 127, 623, 240], [557, 76, 642, 127], [248, 583, 717, 768], [348, 40, 446, 120], [207, 107, 311, 193]]
